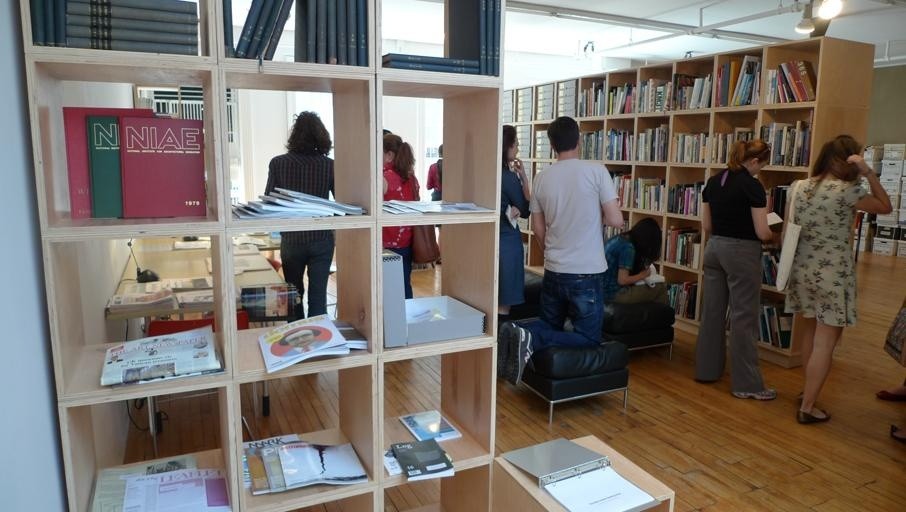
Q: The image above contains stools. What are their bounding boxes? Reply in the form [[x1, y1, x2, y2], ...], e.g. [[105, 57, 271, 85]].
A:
[[599, 296, 675, 358], [504, 314, 629, 422]]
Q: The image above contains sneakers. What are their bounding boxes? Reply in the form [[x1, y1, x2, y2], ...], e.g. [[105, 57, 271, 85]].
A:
[[507, 329, 533, 387], [497, 321, 520, 380], [733, 387, 779, 401]]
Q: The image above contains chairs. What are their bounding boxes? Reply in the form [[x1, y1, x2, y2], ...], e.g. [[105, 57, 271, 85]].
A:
[[853, 211, 865, 262]]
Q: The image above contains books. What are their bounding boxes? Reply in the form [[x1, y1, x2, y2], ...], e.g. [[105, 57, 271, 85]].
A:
[[265, 0, 294, 60], [66, 1, 198, 25], [120, 116, 206, 217], [763, 59, 816, 104], [258, 445, 286, 493], [667, 180, 704, 217], [480, 0, 486, 75], [398, 410, 462, 443], [635, 123, 667, 163], [223, 1, 233, 58], [66, 25, 198, 45], [244, 448, 270, 494], [579, 130, 603, 159], [712, 119, 732, 162], [487, 0, 494, 74], [759, 298, 791, 348], [610, 171, 630, 208], [243, 434, 301, 488], [236, 0, 265, 57], [63, 107, 153, 220], [250, 487, 265, 495], [275, 187, 362, 210], [634, 264, 665, 289], [44, 0, 55, 46], [390, 200, 495, 213], [667, 280, 696, 320], [327, 0, 337, 64], [317, 0, 327, 63], [383, 448, 453, 477], [357, 0, 368, 66], [248, 201, 333, 216], [494, 0, 501, 76], [734, 120, 756, 141], [579, 79, 604, 117], [383, 202, 423, 213], [384, 206, 405, 215], [246, 0, 275, 58], [237, 202, 329, 217], [86, 115, 173, 217], [232, 204, 321, 217], [258, 194, 346, 216], [256, 0, 284, 60], [636, 175, 666, 213], [269, 192, 368, 216], [307, 0, 316, 63], [666, 227, 700, 269], [383, 54, 479, 68], [67, 37, 198, 55], [232, 208, 312, 219], [766, 212, 783, 226], [30, 0, 45, 45], [766, 184, 790, 229], [277, 441, 368, 489], [391, 438, 455, 481], [607, 82, 636, 115], [605, 126, 633, 161], [257, 314, 350, 374], [337, 0, 348, 65], [716, 54, 760, 106], [331, 322, 368, 348], [639, 78, 672, 112], [66, 14, 198, 35], [347, 0, 358, 65], [66, 0, 196, 14], [671, 130, 708, 163], [383, 61, 479, 75], [761, 247, 781, 285], [761, 120, 809, 167], [674, 73, 711, 109], [54, 0, 67, 47]]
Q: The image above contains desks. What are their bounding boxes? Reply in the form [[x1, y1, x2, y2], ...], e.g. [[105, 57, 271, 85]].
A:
[[105, 232, 282, 435]]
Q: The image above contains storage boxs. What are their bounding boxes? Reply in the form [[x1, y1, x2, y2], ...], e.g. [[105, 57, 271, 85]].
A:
[[879, 175, 900, 192], [857, 209, 869, 220], [881, 160, 903, 176], [876, 209, 899, 225], [853, 238, 870, 251], [863, 161, 881, 175], [853, 223, 874, 237], [858, 176, 871, 194], [898, 209, 906, 225], [873, 237, 898, 256], [883, 144, 905, 160], [899, 226, 906, 240], [901, 177, 906, 192], [865, 145, 883, 161], [885, 193, 900, 209], [876, 224, 901, 240], [900, 193, 906, 209], [897, 240, 906, 257]]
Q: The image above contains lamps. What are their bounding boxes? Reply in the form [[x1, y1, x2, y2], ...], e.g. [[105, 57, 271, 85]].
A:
[[795, 4, 815, 34]]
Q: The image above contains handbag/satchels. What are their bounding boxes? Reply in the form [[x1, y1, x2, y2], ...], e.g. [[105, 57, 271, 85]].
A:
[[409, 179, 440, 265], [775, 178, 803, 293]]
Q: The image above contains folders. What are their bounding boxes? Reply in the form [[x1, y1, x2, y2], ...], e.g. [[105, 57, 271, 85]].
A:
[[500, 437, 662, 512]]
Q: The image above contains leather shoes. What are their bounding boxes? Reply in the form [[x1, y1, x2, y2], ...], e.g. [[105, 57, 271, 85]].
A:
[[798, 406, 833, 427], [875, 389, 906, 403], [889, 424, 906, 444]]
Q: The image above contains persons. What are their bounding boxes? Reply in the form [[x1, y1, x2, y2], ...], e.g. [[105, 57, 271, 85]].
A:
[[498, 125, 530, 334], [876, 299, 906, 444], [691, 140, 776, 400], [383, 133, 420, 299], [497, 116, 625, 385], [782, 135, 894, 425], [264, 111, 335, 323], [601, 217, 668, 304], [426, 144, 443, 264]]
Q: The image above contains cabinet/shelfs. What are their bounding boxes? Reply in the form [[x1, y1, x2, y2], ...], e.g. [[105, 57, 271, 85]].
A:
[[503, 34, 875, 368], [16, 0, 507, 512], [493, 434, 675, 511]]
